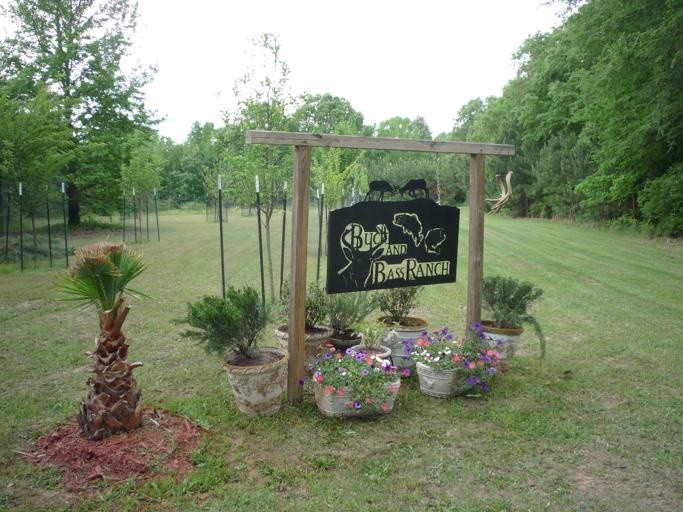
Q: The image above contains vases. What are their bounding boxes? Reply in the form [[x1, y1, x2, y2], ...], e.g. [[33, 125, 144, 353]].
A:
[[415, 355, 483, 398], [309, 370, 401, 420]]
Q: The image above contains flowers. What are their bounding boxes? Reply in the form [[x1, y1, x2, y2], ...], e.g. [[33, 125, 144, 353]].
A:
[[405, 323, 506, 408], [298, 339, 408, 422]]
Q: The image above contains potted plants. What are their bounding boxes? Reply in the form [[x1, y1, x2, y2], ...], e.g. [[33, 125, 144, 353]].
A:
[[270, 275, 429, 378], [171, 282, 290, 418], [476, 271, 546, 364]]
